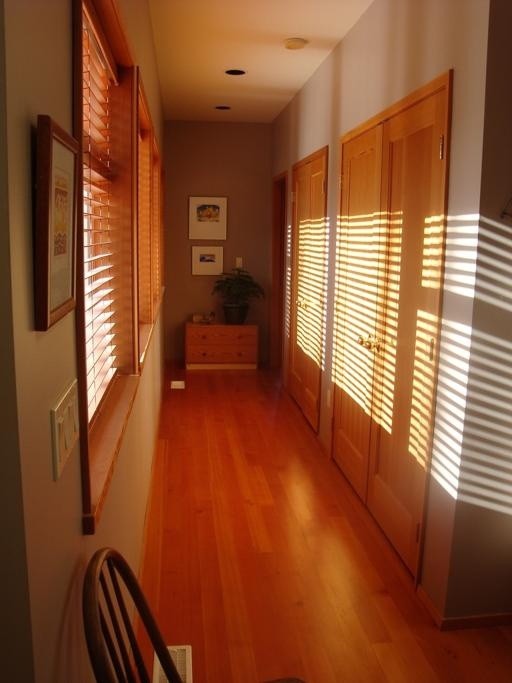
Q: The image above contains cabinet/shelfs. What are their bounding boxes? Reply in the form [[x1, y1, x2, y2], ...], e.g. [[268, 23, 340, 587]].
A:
[[182, 321, 260, 377]]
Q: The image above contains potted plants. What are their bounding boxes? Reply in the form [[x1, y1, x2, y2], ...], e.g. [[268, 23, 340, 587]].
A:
[[209, 268, 265, 325]]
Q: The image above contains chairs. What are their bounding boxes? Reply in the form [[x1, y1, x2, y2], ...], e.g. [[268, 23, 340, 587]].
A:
[[82, 545, 303, 683]]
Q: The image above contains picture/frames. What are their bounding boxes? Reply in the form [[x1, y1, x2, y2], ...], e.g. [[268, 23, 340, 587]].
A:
[[191, 245, 224, 276], [31, 112, 81, 331], [188, 196, 228, 241]]
